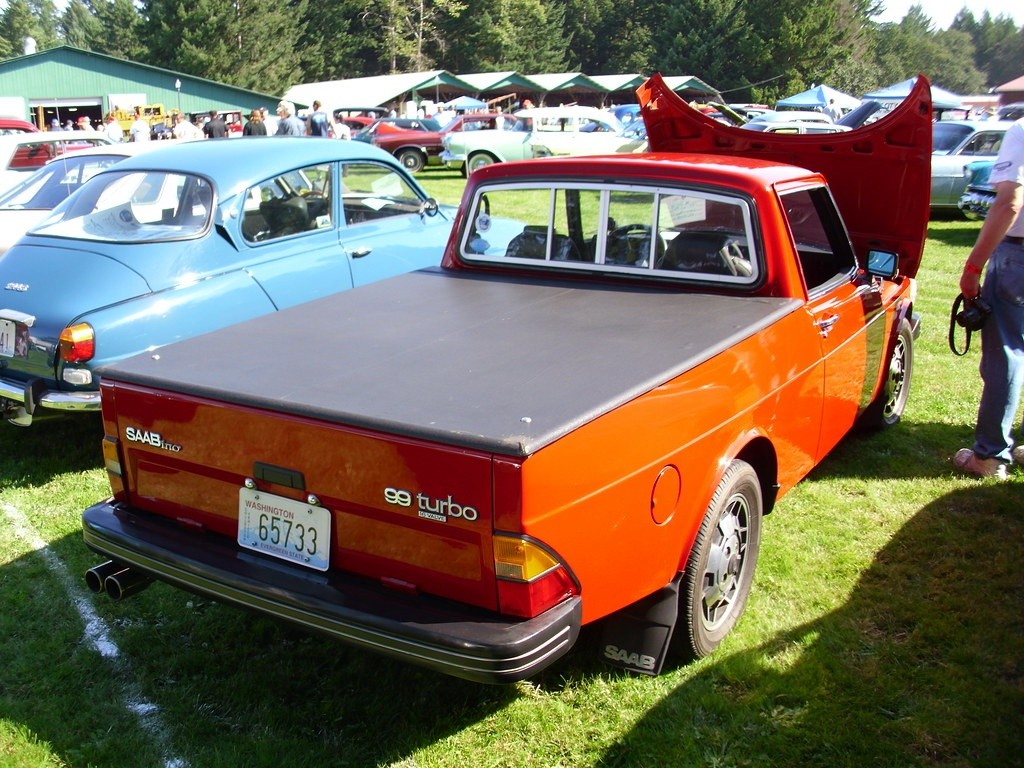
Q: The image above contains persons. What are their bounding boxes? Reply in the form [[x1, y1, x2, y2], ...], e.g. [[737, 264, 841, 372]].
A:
[[51, 100, 351, 178], [432, 102, 455, 126], [495, 106, 504, 128], [514, 100, 533, 126], [955, 115, 1024, 480], [823, 98, 842, 122], [815, 107, 824, 123]]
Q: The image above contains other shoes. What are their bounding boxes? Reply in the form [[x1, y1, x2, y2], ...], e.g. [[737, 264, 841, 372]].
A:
[[1012, 445, 1023, 464], [955, 448, 1007, 480]]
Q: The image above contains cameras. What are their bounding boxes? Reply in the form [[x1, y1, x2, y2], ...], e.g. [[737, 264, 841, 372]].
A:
[[957, 297, 993, 332]]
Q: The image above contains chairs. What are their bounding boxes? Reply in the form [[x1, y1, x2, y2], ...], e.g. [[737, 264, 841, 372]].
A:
[[654, 229, 752, 277], [189, 188, 212, 225], [259, 197, 312, 240], [505, 230, 582, 263]]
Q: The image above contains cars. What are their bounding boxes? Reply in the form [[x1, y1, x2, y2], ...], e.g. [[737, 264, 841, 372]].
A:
[[0, 102, 1024, 290], [0, 135, 529, 427]]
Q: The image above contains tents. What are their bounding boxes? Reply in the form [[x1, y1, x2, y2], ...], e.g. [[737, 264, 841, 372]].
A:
[[775, 85, 861, 111], [863, 76, 960, 107], [444, 96, 488, 114]]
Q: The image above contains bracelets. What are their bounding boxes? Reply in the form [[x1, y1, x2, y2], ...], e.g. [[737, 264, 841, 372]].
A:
[[965, 263, 981, 274]]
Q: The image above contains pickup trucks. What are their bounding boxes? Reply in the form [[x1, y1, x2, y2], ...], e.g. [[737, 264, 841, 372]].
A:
[[79, 69, 938, 689]]
